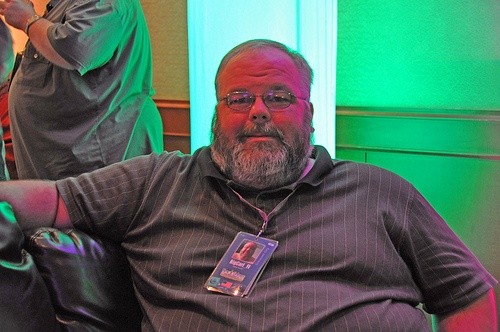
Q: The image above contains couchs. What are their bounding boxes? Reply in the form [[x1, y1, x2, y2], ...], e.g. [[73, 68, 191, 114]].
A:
[[0, 202, 141, 332]]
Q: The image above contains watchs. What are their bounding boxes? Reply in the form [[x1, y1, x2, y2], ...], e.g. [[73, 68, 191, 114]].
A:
[[25, 14, 42, 37]]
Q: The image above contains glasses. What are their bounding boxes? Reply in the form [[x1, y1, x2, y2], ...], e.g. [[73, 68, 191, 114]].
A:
[[219, 88, 307, 114]]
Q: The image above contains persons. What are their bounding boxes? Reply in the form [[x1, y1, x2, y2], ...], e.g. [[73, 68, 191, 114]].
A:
[[0, 39, 498, 332], [0, 0, 165, 181], [234, 242, 257, 262]]
